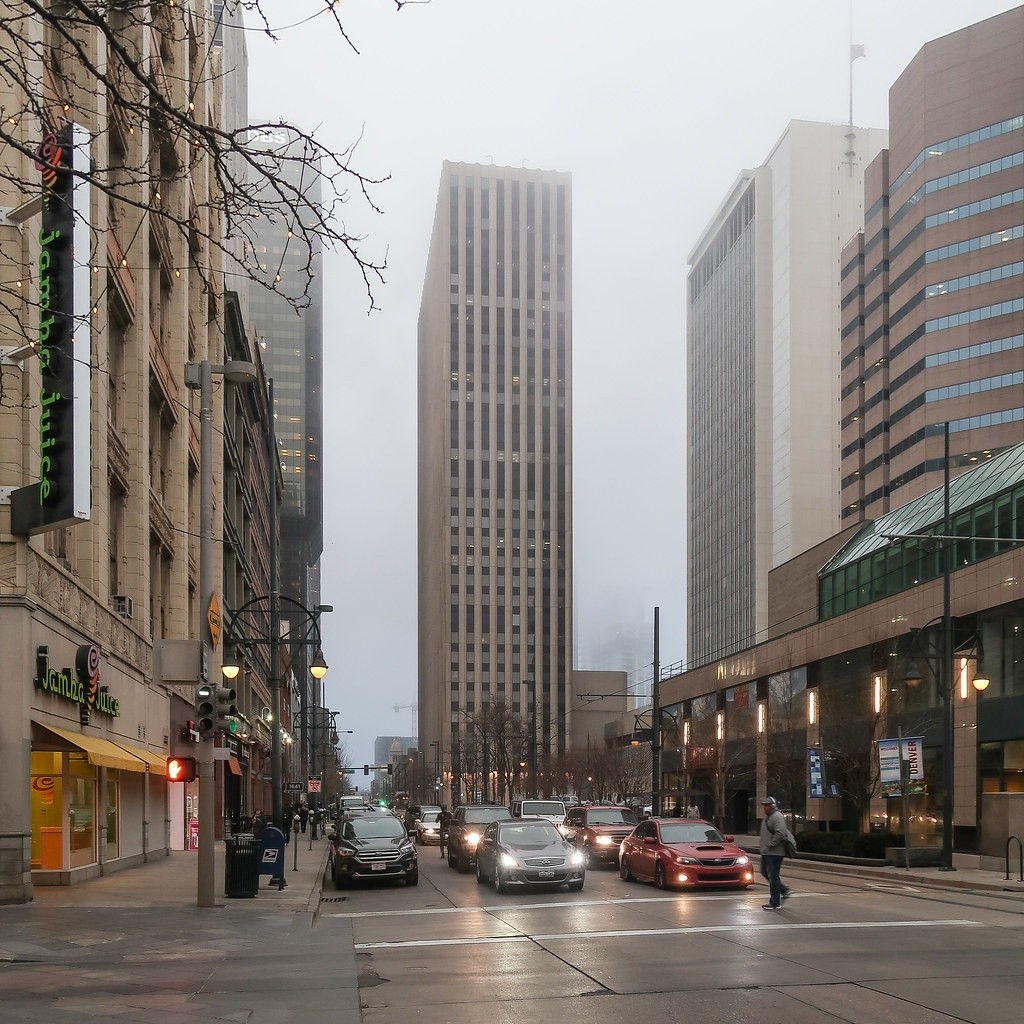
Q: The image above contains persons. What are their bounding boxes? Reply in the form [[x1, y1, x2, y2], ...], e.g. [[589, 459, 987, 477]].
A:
[[687, 801, 700, 819], [247, 809, 267, 833], [435, 806, 453, 858], [673, 801, 681, 817], [759, 797, 795, 910], [283, 799, 320, 846]]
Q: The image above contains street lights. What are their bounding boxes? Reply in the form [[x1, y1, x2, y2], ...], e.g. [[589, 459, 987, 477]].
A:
[[220, 595, 328, 892], [432, 740, 440, 805], [430, 743, 436, 805], [905, 616, 991, 866], [631, 708, 678, 822]]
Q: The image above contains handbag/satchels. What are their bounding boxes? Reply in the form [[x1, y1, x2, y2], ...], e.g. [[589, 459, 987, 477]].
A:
[[783, 829, 798, 858]]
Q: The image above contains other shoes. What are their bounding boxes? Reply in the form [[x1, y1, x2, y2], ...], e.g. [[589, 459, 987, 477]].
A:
[[762, 904, 781, 910], [440, 852, 444, 858], [783, 890, 794, 899]]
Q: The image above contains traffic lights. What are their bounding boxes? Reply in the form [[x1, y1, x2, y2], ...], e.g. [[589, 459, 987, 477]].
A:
[[194, 684, 218, 735], [165, 756, 197, 783], [216, 685, 238, 735]]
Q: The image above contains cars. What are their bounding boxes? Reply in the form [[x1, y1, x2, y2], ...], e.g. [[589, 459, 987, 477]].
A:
[[560, 793, 677, 819], [336, 796, 389, 810], [618, 817, 755, 889], [414, 811, 450, 846], [474, 818, 586, 891]]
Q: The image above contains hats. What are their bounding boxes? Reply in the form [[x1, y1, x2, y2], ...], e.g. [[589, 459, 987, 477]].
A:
[[760, 797, 776, 804]]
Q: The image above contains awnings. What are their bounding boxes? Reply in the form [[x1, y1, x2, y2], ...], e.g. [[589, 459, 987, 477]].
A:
[[32, 720, 170, 776]]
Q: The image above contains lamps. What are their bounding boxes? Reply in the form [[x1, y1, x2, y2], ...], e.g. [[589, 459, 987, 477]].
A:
[[808, 691, 816, 727], [960, 659, 969, 699], [282, 734, 291, 743], [873, 674, 882, 715], [281, 727, 288, 739], [717, 713, 723, 740], [758, 703, 764, 733], [261, 707, 272, 722], [683, 721, 690, 746]]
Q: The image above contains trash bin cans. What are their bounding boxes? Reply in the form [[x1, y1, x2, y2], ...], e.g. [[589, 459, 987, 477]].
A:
[[221, 833, 263, 898], [258, 827, 286, 891]]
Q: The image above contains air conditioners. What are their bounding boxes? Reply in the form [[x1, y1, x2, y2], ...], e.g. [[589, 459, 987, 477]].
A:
[[111, 595, 134, 619]]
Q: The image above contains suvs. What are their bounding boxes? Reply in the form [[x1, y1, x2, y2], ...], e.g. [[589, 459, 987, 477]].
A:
[[561, 804, 643, 868], [512, 800, 572, 840], [403, 803, 442, 835], [444, 805, 517, 875], [326, 811, 420, 890]]
